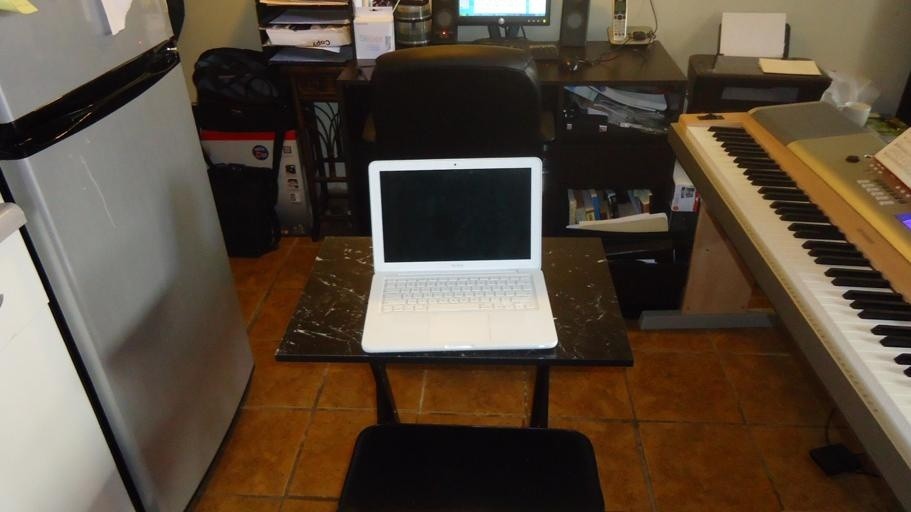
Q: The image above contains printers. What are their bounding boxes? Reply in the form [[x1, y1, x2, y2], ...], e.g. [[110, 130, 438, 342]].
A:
[[688, 24, 833, 117]]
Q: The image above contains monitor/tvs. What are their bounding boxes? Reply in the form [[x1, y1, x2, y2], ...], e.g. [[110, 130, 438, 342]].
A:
[[456, 0, 551, 40]]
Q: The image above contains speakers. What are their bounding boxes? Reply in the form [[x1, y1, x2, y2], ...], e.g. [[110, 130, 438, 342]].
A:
[[559, 0, 590, 47], [430, 0, 459, 45]]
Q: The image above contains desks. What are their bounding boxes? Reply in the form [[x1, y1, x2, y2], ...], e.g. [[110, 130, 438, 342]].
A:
[[335, 33, 690, 250], [284, 61, 355, 241], [273, 231, 638, 512]]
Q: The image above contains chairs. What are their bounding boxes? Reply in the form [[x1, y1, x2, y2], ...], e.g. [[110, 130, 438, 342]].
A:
[[359, 39, 574, 241]]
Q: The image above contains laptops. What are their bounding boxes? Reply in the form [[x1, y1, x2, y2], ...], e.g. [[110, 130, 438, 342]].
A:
[[361, 156, 559, 354]]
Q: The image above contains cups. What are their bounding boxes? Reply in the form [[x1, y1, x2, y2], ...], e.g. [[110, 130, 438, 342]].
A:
[[838, 101, 871, 127]]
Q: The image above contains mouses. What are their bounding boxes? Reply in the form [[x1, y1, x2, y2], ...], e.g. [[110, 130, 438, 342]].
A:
[[561, 56, 577, 72]]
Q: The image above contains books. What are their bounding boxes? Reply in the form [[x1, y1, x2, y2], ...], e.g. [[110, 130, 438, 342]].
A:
[[567, 188, 670, 232], [564, 83, 668, 135]]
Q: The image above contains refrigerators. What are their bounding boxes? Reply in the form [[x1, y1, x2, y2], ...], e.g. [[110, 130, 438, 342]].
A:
[[2, 3, 256, 511]]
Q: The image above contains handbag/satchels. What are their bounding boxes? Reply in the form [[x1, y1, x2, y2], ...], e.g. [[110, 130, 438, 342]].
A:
[[193, 47, 294, 132], [208, 162, 280, 258]]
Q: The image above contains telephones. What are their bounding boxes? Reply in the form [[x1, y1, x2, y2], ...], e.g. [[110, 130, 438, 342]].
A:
[[607, 0, 653, 49]]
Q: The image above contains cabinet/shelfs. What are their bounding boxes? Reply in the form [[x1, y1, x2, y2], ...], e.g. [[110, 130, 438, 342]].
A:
[[0, 201, 137, 511]]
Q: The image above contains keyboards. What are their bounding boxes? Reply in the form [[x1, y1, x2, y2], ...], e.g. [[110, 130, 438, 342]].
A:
[[528, 47, 558, 61]]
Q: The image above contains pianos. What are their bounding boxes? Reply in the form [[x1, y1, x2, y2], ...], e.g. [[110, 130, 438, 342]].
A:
[[639, 100, 910, 511]]
